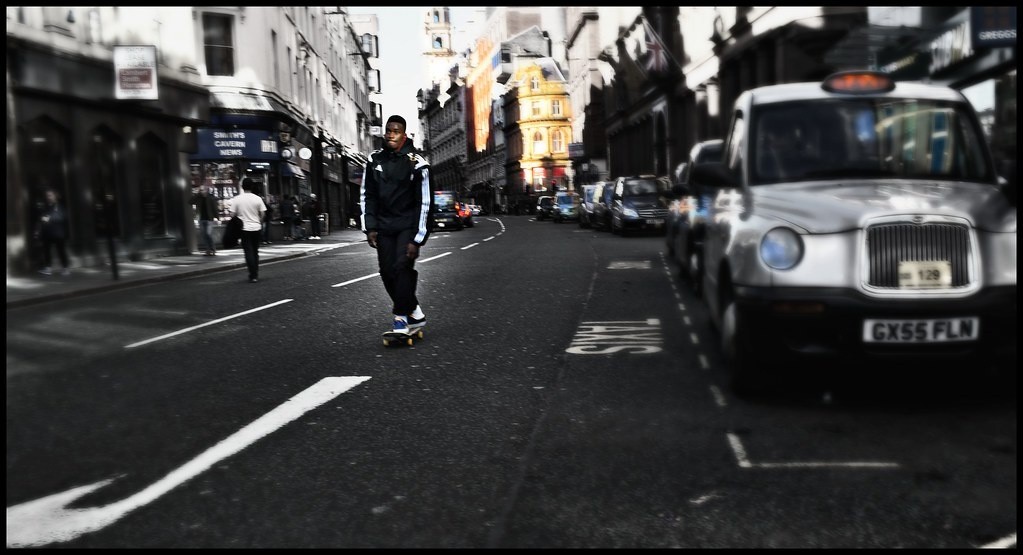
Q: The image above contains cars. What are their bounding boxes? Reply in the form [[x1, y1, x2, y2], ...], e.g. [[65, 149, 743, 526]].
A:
[[431, 190, 462, 230], [457, 202, 474, 228], [694, 70, 1020, 382], [667, 139, 728, 277], [468, 205, 479, 216], [535, 174, 671, 235]]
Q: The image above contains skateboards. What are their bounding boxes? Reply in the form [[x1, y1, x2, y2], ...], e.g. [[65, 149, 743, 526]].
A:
[[381, 318, 428, 347]]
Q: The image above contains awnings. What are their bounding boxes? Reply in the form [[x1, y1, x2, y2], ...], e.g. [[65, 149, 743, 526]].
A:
[[284, 163, 306, 180]]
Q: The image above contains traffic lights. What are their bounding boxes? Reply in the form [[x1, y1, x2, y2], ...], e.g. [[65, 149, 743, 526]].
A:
[[551, 179, 557, 192]]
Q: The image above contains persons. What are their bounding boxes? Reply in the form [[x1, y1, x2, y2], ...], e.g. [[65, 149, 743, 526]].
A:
[[34, 188, 75, 276], [194, 185, 220, 257], [228, 179, 269, 283], [361, 115, 433, 335], [261, 193, 322, 245]]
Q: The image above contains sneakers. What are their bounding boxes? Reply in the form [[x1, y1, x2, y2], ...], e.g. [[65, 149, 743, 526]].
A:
[[392, 317, 410, 334], [408, 315, 427, 328]]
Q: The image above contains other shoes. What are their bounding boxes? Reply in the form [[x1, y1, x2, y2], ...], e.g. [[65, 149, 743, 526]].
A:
[[37, 270, 52, 275], [250, 275, 258, 283], [202, 249, 215, 256], [61, 271, 72, 277]]
